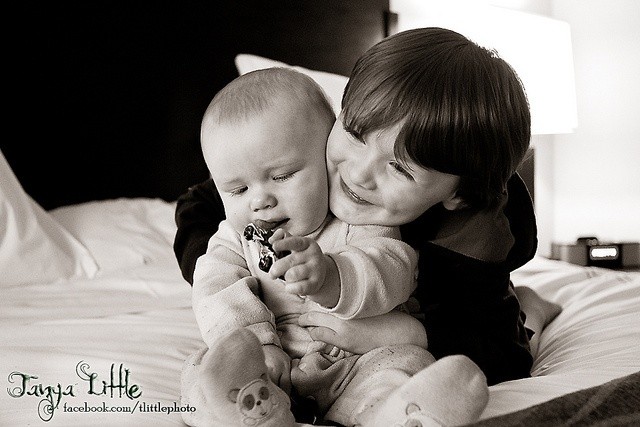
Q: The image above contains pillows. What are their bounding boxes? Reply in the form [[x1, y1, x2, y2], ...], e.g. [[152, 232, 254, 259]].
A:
[[233, 51, 368, 130], [1, 150, 101, 284]]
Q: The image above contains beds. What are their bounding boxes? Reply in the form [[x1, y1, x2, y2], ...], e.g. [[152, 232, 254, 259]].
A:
[[1, 1, 639, 427]]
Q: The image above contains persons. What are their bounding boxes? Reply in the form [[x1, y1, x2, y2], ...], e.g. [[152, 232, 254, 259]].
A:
[[178, 67, 489, 427], [174, 26, 564, 387], [240, 220, 292, 270]]
